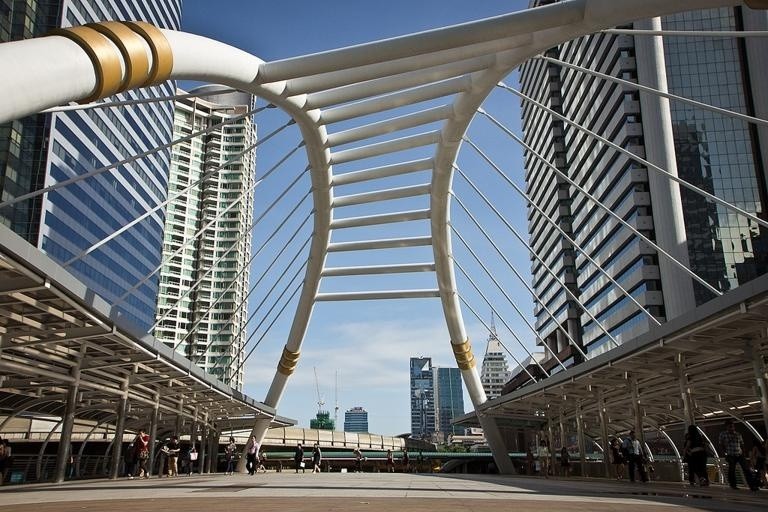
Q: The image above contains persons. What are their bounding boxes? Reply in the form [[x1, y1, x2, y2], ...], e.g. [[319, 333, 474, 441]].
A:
[[127, 429, 196, 480], [0, 437, 11, 486], [312, 444, 322, 473], [387, 449, 448, 473], [354, 448, 362, 472], [537, 421, 768, 492], [295, 444, 305, 473], [224, 435, 266, 475]]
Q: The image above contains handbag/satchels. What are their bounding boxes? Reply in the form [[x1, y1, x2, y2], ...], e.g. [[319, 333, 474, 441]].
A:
[[190, 452, 198, 460], [300, 462, 305, 467]]
